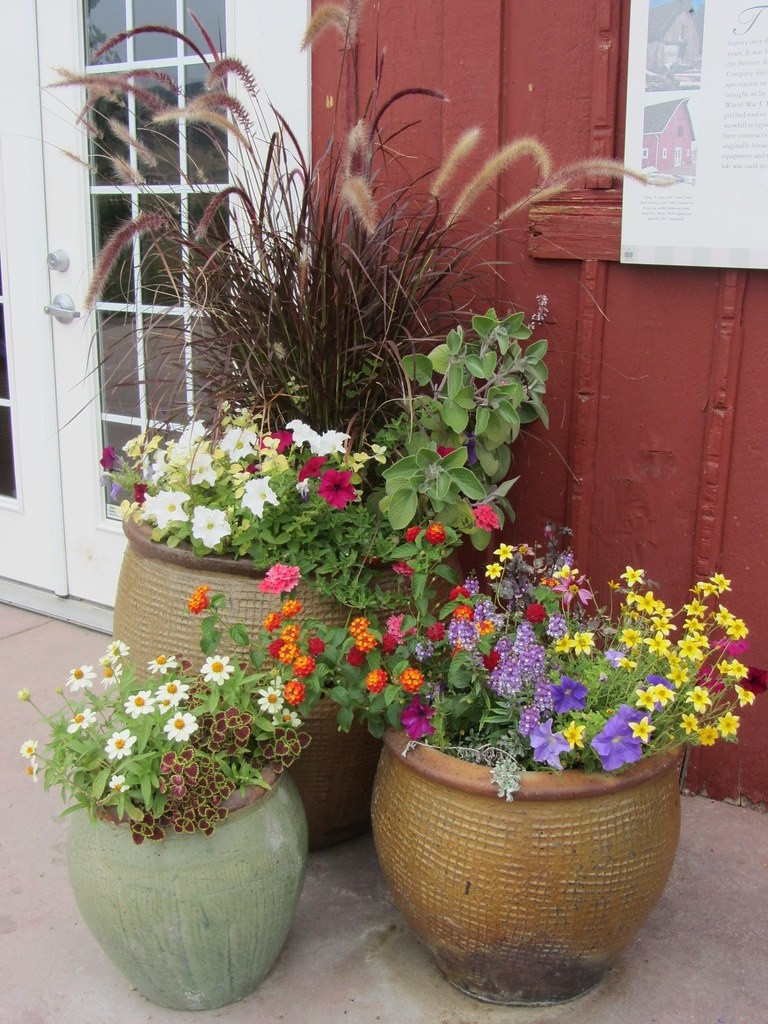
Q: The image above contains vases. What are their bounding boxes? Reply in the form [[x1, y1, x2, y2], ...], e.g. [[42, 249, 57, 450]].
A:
[[61, 760, 312, 1014], [109, 506, 467, 848], [371, 721, 689, 1009]]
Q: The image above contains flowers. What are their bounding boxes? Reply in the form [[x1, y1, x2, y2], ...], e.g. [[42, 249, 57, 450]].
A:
[[15, 639, 314, 845], [95, 298, 556, 607], [186, 520, 767, 805]]
